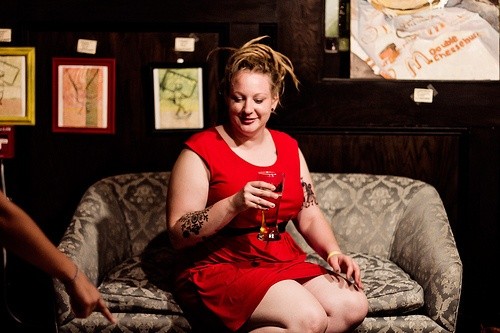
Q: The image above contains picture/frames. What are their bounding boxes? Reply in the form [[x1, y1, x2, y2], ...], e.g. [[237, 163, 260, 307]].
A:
[[51, 58, 115, 134], [0, 47, 36, 126]]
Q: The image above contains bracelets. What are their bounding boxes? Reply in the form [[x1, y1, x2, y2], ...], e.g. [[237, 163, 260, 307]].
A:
[[71, 262, 80, 285], [327, 251, 340, 263]]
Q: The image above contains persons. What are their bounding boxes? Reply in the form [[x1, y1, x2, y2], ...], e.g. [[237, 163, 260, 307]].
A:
[[0, 190, 116, 327], [167, 35, 369, 333]]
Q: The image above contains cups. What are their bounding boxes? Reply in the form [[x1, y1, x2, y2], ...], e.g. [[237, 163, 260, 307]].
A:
[[258, 170, 282, 241]]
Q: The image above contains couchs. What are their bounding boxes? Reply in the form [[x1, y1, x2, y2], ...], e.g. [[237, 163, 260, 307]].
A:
[[53, 173, 463, 333]]
